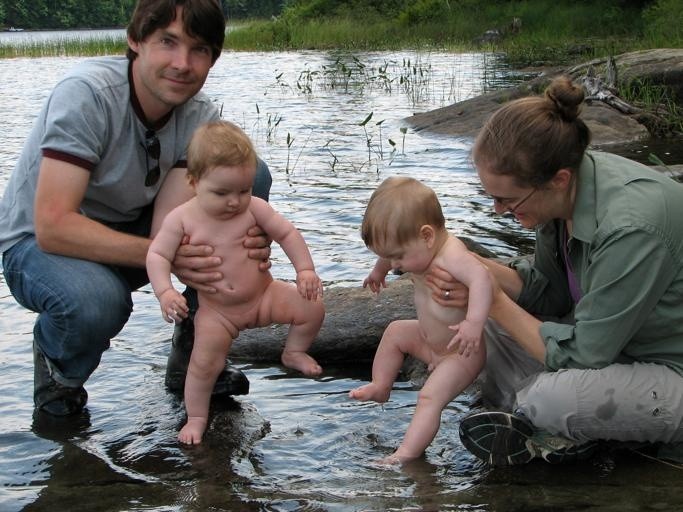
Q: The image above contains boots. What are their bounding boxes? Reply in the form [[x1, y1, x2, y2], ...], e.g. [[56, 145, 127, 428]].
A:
[[163, 312, 250, 396], [33, 338, 88, 416]]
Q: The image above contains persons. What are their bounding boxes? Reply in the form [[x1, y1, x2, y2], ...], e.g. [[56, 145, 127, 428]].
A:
[[422, 76, 683, 471], [346, 176, 494, 467], [0, 1, 274, 418], [144, 120, 325, 445]]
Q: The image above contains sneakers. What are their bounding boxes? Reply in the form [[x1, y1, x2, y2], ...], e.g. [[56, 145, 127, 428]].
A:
[[458, 412, 601, 467]]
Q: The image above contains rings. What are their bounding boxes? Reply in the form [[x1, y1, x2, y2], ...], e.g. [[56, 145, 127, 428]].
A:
[[444, 290, 451, 300]]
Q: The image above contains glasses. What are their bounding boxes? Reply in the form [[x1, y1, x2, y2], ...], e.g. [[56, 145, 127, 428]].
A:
[[496, 186, 535, 216], [139, 131, 161, 188]]
